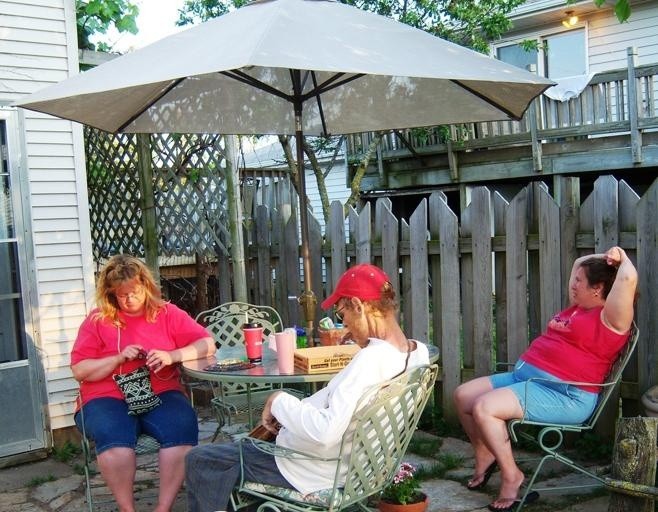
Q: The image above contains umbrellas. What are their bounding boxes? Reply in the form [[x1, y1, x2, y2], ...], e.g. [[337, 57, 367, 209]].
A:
[[11, 0, 562, 396]]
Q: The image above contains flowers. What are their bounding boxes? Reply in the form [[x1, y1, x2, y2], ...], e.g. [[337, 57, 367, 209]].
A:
[[385, 463, 423, 502]]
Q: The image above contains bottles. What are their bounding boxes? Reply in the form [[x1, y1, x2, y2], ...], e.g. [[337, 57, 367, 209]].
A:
[[297, 328, 306, 348]]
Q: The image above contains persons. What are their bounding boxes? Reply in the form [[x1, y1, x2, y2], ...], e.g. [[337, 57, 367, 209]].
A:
[[66, 252, 219, 511], [451, 244, 639, 509], [177, 264, 430, 509]]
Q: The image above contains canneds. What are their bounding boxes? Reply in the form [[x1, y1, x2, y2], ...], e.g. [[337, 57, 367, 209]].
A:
[[318, 316, 335, 329]]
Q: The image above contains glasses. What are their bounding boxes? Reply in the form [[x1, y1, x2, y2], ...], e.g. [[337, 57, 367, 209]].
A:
[[335, 302, 351, 321]]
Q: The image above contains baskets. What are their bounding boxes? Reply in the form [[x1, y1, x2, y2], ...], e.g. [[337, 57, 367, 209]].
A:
[[317, 326, 350, 346]]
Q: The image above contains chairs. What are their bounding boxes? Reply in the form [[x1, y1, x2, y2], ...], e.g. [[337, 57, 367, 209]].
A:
[[492, 319, 640, 510], [226, 363, 439, 510], [195, 302, 312, 442], [74, 382, 194, 510]]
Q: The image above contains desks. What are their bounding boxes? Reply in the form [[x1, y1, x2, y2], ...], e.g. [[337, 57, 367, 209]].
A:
[[181, 337, 439, 436]]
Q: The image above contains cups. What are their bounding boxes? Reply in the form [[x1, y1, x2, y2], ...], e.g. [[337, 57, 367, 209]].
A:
[[241, 322, 264, 365], [273, 332, 296, 374]]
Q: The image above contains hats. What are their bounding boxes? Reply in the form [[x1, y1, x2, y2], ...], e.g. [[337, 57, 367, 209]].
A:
[[320, 264, 395, 311]]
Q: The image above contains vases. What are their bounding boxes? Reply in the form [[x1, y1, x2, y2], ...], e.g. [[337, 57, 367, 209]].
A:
[[377, 490, 428, 510]]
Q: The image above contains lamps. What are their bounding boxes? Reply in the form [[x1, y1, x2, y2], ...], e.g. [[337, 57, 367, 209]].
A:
[[562, 10, 579, 28]]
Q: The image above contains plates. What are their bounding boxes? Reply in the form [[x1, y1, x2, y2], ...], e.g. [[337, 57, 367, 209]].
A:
[[203, 357, 256, 372]]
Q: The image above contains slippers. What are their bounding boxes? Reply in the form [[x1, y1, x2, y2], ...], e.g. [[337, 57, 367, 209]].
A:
[[468, 460, 496, 490], [488, 491, 539, 512]]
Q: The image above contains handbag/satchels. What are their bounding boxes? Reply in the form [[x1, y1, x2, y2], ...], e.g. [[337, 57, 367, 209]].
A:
[[113, 364, 162, 416]]
